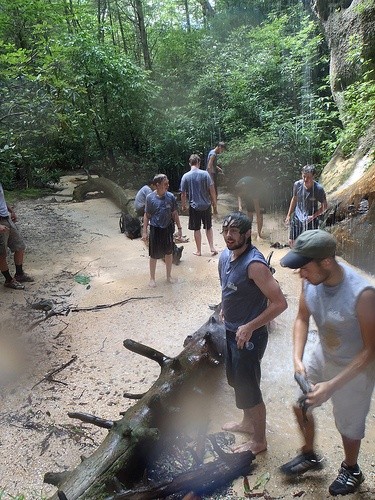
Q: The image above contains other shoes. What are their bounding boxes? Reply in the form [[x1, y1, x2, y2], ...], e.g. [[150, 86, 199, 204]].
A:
[[173, 234, 187, 238], [175, 237, 190, 243]]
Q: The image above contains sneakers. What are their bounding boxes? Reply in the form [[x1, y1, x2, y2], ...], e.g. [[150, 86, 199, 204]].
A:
[[4, 278, 24, 289], [278, 451, 319, 477], [13, 272, 34, 282], [329, 463, 365, 496]]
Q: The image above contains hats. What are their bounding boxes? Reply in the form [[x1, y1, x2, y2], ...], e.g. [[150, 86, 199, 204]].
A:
[[279, 229, 337, 269]]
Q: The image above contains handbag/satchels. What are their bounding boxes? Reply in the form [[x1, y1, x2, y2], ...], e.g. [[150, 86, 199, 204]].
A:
[[122, 212, 141, 238]]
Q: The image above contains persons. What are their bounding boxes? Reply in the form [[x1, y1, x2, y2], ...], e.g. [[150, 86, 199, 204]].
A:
[[134, 178, 158, 217], [235, 176, 268, 242], [279, 229, 375, 496], [0, 182, 34, 289], [218, 211, 289, 455], [140, 174, 183, 288], [179, 154, 218, 256], [205, 142, 227, 199], [284, 163, 328, 250]]
